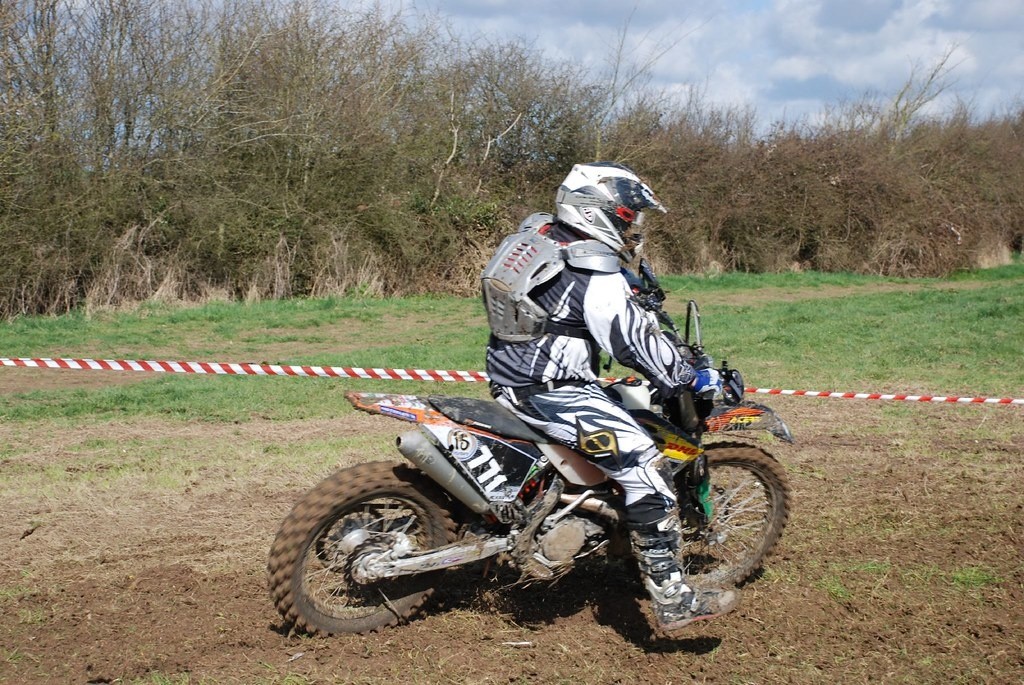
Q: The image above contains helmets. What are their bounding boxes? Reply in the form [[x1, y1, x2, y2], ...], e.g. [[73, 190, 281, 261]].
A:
[[555, 161, 668, 263]]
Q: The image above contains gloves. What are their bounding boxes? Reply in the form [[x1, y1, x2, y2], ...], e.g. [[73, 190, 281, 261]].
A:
[[687, 368, 722, 399]]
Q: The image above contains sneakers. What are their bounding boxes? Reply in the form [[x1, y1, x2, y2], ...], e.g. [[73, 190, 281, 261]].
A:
[[655, 583, 737, 631]]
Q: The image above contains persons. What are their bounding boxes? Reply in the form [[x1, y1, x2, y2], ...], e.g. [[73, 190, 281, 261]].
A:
[[485, 163, 743, 631]]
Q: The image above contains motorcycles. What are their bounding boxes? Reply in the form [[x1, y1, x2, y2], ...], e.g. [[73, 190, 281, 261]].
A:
[[265, 258, 795, 638]]
